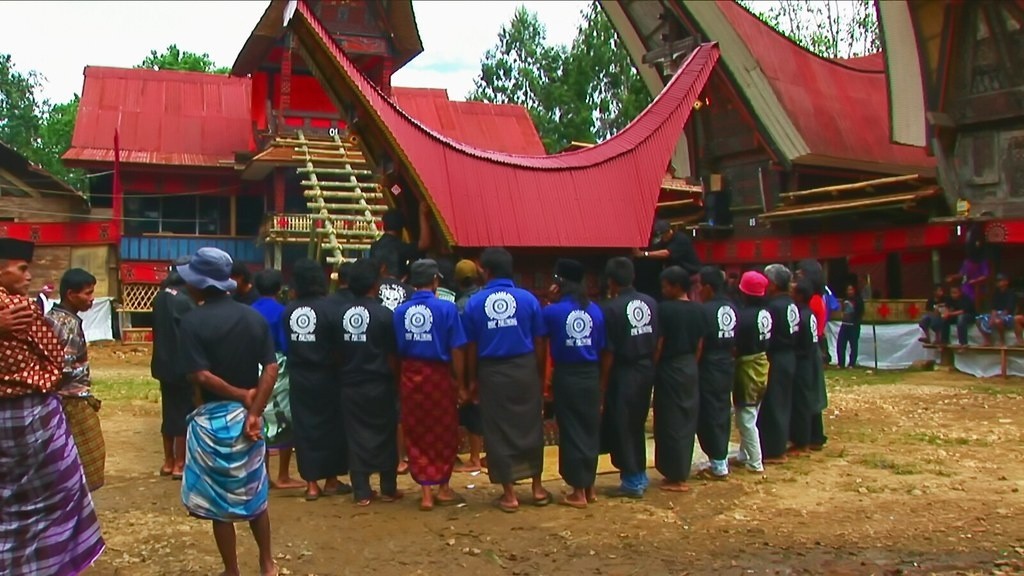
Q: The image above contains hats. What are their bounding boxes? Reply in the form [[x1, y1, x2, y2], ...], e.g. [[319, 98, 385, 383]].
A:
[[0, 238, 34, 261], [42, 283, 54, 296], [412, 258, 446, 285], [739, 271, 769, 296], [452, 259, 478, 280], [993, 272, 1012, 282], [653, 220, 671, 245], [170, 247, 238, 292]]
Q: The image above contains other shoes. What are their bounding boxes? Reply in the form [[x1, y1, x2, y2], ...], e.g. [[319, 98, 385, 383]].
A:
[[606, 486, 647, 498], [481, 455, 490, 474]]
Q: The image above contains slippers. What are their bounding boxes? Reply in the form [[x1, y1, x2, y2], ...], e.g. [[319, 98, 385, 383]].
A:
[[586, 498, 597, 503], [491, 495, 517, 512], [382, 492, 405, 502], [728, 457, 746, 468], [323, 482, 352, 495], [160, 471, 171, 477], [560, 495, 589, 509], [304, 485, 320, 500], [419, 495, 436, 511], [359, 490, 378, 506], [435, 498, 466, 506], [172, 475, 182, 480], [786, 447, 811, 457], [399, 460, 410, 475], [534, 488, 553, 506]]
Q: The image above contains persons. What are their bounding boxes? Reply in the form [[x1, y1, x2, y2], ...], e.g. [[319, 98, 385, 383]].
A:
[[0, 238, 107, 576], [916, 247, 1024, 348], [151, 202, 862, 576]]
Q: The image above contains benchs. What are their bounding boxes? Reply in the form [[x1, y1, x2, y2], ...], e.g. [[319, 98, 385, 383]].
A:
[[923, 343, 1024, 380]]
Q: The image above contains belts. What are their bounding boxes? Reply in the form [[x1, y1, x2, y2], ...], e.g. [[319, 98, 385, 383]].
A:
[[0, 394, 43, 411]]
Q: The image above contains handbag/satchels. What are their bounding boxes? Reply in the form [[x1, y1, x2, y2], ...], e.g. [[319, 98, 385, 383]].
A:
[[54, 391, 105, 492]]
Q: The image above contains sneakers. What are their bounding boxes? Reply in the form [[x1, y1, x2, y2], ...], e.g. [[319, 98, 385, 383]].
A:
[[695, 467, 732, 481]]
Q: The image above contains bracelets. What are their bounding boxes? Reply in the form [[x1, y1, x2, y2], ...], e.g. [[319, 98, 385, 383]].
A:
[[645, 251, 648, 258]]
